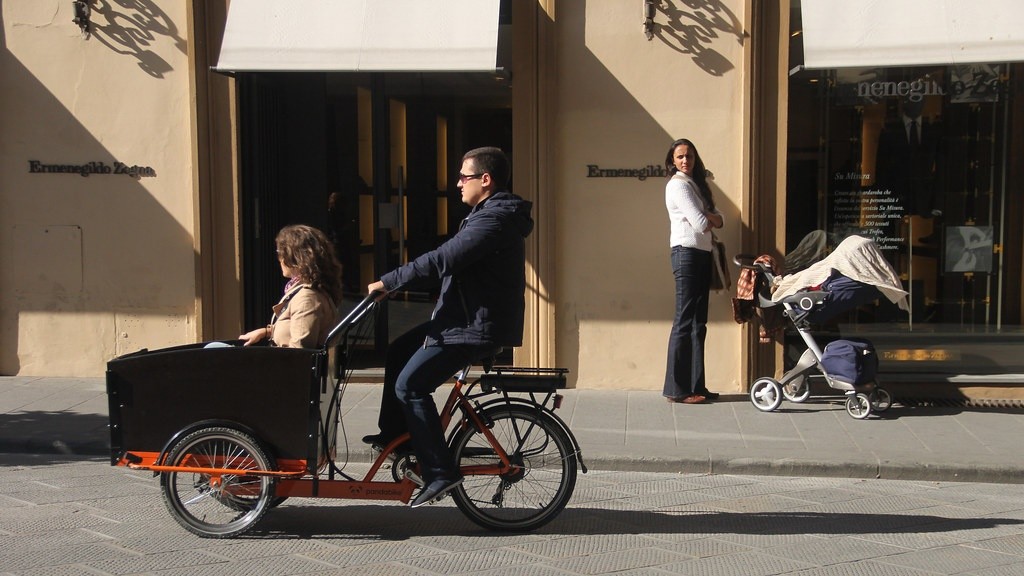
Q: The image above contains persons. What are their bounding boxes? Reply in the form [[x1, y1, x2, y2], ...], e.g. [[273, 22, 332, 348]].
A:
[[662, 138, 723, 406], [367, 146, 535, 510], [238, 225, 344, 352]]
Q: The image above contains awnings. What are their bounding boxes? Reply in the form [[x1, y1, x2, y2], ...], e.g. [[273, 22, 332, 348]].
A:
[[789, 0, 1024, 77], [209, 0, 512, 79]]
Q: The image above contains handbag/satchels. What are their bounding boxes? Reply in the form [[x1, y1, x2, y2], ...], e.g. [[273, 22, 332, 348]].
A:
[[708, 242, 731, 290], [821, 335, 878, 385]]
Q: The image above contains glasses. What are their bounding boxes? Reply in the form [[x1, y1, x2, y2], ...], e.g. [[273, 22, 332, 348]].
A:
[[458, 171, 484, 184]]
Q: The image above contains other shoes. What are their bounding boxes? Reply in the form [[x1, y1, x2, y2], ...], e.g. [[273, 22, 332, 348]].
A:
[[693, 387, 719, 400], [667, 393, 706, 404], [410, 473, 465, 508], [362, 432, 400, 448]]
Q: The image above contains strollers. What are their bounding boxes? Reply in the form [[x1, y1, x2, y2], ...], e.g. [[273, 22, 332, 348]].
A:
[[734, 235, 903, 420]]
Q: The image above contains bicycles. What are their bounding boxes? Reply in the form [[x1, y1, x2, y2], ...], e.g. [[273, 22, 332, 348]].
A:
[[106, 289, 589, 540]]
